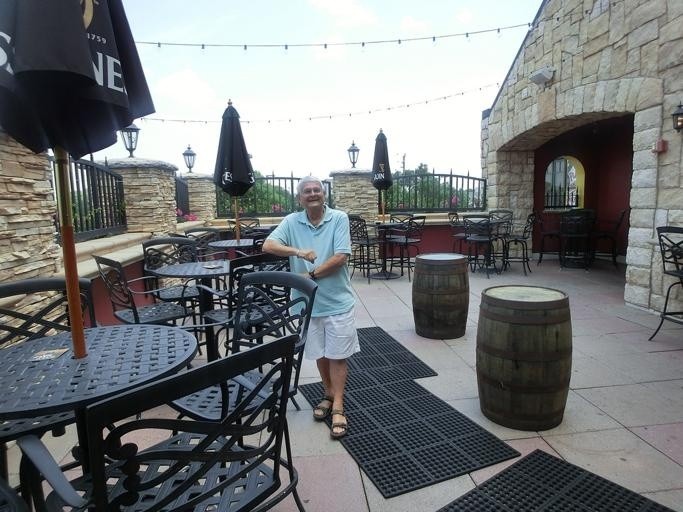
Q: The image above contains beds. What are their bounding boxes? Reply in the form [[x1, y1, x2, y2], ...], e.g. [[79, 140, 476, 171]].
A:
[[0, 1, 155, 358], [369, 128, 394, 218], [210, 97, 255, 243]]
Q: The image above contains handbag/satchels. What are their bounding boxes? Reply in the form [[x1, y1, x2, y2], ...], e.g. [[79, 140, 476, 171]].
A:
[[296, 247, 301, 259], [308, 269, 315, 281]]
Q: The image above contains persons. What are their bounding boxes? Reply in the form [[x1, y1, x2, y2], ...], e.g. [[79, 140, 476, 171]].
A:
[[262, 176, 361, 440]]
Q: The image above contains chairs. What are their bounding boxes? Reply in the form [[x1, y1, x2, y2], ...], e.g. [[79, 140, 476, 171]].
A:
[[0, 218, 318, 511], [648, 226, 683, 341], [349, 206, 629, 284]]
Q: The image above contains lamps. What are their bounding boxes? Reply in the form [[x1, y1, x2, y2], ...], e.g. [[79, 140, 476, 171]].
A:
[[670, 101, 683, 132], [349, 206, 629, 284], [648, 226, 683, 341]]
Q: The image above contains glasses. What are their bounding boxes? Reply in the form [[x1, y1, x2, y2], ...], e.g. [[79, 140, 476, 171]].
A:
[[670, 101, 683, 132]]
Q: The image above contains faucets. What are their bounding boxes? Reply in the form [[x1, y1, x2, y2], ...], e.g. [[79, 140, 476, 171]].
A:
[[330, 409, 348, 438], [313, 395, 334, 419]]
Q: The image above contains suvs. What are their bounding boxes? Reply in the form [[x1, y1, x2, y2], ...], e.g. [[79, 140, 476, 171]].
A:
[[476, 285, 572, 429], [412, 253, 467, 339]]
